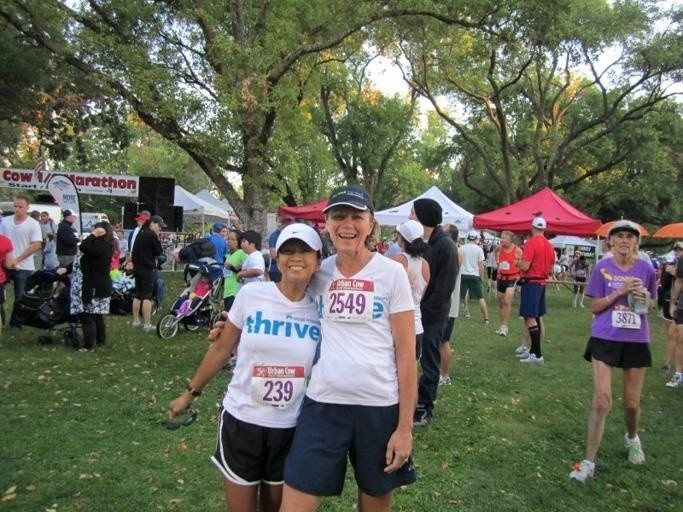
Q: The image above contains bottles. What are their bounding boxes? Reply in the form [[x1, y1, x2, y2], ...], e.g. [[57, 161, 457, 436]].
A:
[[635, 300, 648, 313]]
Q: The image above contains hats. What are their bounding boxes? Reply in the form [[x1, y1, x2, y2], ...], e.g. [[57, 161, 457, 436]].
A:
[[213, 223, 222, 230], [468, 231, 478, 239], [322, 185, 375, 218], [414, 198, 443, 228], [151, 216, 168, 228], [532, 217, 547, 229], [608, 220, 640, 238], [396, 219, 425, 244], [90, 213, 109, 225], [275, 222, 323, 257], [135, 211, 151, 222]]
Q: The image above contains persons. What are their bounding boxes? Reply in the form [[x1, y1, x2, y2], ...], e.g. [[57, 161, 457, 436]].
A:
[[365, 199, 557, 424], [0, 194, 167, 352], [207, 188, 418, 512], [165, 222, 326, 511], [171, 214, 298, 313], [568, 219, 660, 480], [568, 242, 683, 388]]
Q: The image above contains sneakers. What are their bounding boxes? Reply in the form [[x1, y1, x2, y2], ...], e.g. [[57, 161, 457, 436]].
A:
[[79, 347, 95, 354], [666, 372, 682, 388], [439, 375, 452, 386], [495, 326, 508, 337], [625, 431, 646, 465], [413, 410, 434, 425], [569, 460, 595, 484], [133, 321, 156, 332], [515, 346, 545, 364]]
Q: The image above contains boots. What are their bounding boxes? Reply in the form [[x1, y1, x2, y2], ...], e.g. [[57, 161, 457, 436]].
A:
[[572, 293, 585, 309]]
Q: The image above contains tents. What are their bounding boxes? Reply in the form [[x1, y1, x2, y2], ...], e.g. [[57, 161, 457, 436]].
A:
[[374, 185, 473, 234], [172, 183, 239, 241], [472, 187, 606, 274], [277, 196, 333, 232]]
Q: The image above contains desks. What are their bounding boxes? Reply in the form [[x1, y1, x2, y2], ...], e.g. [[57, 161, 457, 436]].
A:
[[548, 280, 589, 286]]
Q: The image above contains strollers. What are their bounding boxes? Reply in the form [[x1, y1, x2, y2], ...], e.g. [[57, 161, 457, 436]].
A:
[[157, 261, 238, 339], [12, 267, 82, 347]]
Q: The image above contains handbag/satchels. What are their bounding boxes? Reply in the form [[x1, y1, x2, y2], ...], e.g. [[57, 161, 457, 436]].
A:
[[516, 276, 530, 287]]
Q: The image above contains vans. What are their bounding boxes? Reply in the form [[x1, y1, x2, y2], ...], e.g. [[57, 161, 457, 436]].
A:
[[81, 213, 110, 232], [0, 201, 62, 234]]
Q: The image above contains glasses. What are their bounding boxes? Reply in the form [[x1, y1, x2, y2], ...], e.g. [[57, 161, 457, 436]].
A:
[[161, 407, 198, 430]]
